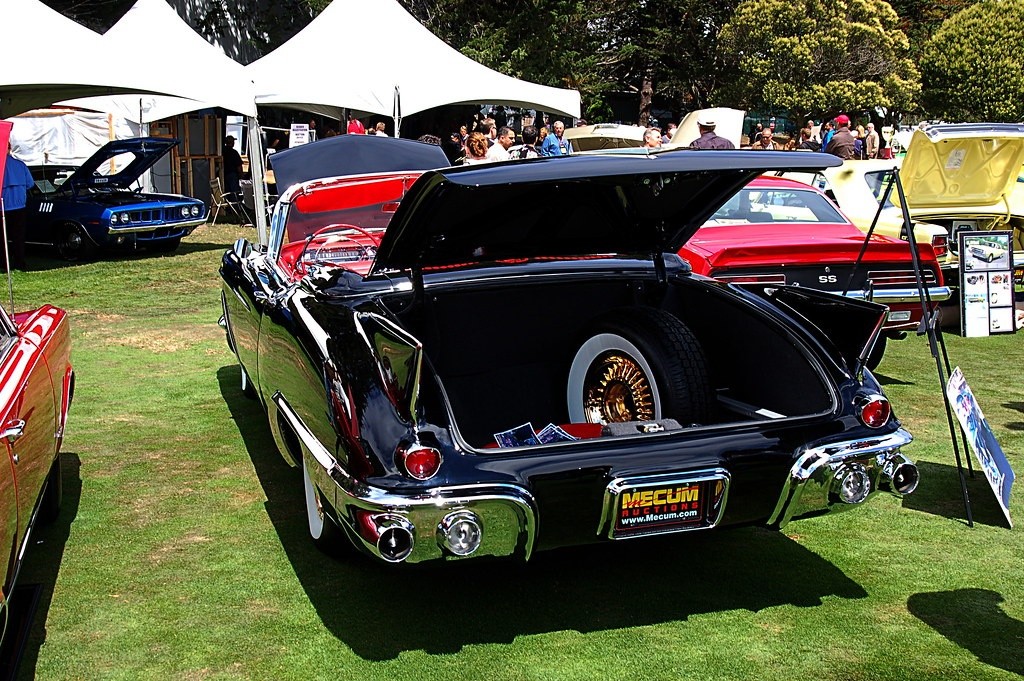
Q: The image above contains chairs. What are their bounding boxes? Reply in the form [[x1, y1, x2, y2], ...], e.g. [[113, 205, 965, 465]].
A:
[[204, 177, 244, 226], [239, 179, 274, 228]]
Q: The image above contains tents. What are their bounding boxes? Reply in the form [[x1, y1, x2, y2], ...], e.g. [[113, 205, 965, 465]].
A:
[[0, 0, 582, 248]]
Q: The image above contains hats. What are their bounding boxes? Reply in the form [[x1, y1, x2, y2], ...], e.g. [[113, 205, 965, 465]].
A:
[[696, 113, 717, 126], [225, 135, 237, 140], [834, 114, 849, 124]]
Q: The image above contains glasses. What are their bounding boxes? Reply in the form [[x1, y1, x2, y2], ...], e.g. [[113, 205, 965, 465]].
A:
[[762, 135, 771, 138]]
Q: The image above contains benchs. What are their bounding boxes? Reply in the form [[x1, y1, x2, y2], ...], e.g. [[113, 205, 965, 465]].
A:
[[710, 212, 773, 223]]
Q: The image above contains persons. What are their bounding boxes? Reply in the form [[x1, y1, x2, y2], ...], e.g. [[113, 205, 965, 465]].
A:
[[1, 141, 36, 271], [212, 105, 932, 233]]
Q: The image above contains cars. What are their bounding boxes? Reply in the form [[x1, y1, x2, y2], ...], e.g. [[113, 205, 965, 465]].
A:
[[0, 302, 76, 635], [218, 130, 919, 569], [752, 124, 1024, 298], [20, 134, 212, 255], [563, 107, 747, 150], [675, 176, 952, 371]]
